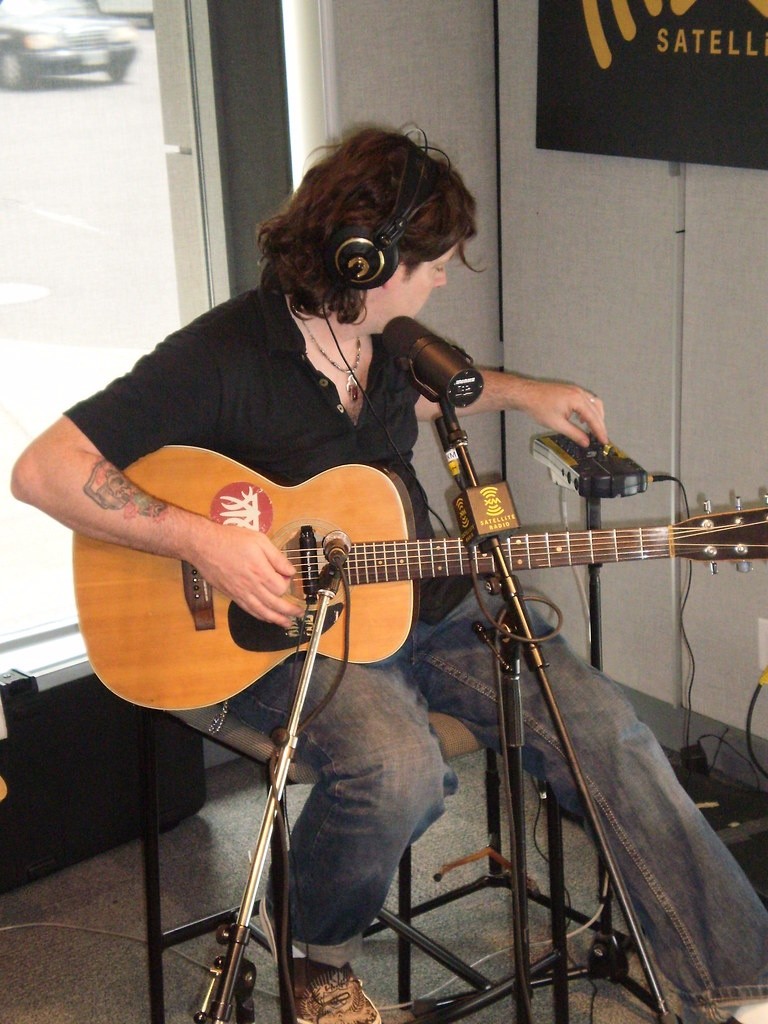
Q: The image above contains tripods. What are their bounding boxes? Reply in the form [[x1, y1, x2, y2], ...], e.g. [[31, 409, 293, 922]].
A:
[[357, 400, 683, 1024]]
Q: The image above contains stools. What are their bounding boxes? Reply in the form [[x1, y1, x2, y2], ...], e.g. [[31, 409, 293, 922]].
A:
[[141, 702, 571, 1023]]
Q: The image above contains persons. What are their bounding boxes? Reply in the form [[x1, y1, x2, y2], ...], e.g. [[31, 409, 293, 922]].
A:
[[10, 129, 768, 1024]]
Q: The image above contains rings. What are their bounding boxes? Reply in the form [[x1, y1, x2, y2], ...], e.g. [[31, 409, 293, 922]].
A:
[[589, 397, 596, 404]]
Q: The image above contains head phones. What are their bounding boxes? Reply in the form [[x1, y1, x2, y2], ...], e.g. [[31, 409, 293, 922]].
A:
[[326, 129, 451, 289]]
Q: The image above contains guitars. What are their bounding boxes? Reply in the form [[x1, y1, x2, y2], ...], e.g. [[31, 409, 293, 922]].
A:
[[74, 445, 768, 712]]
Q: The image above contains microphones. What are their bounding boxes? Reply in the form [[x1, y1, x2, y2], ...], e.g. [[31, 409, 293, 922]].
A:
[[322, 529, 352, 566], [382, 316, 484, 407]]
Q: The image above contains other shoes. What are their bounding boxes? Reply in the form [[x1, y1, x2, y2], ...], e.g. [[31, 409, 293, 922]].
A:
[[259, 894, 383, 1024]]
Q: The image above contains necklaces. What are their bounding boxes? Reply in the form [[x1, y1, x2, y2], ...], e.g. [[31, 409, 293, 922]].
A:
[[290, 300, 361, 404]]
